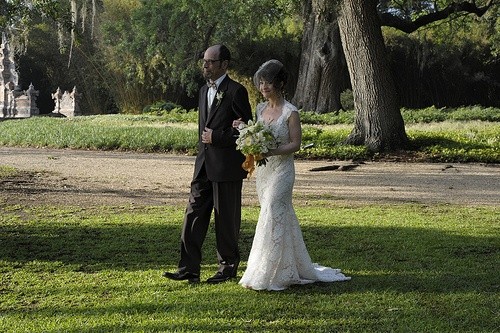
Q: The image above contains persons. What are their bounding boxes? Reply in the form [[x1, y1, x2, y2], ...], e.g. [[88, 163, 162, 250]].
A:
[[232, 60, 352, 291], [162, 44, 253, 283]]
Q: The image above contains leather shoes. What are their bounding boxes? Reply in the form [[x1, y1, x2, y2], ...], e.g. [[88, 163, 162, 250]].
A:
[[163, 270, 200, 284], [207, 272, 236, 284]]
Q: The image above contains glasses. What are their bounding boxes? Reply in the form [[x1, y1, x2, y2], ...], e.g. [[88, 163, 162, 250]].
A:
[[203, 58, 225, 65]]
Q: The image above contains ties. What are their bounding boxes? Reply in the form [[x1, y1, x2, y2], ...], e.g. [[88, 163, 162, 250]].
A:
[[207, 81, 217, 90]]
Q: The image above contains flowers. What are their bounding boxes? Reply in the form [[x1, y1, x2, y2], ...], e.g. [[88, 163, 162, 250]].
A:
[[215, 91, 224, 107], [233, 118, 282, 183]]
